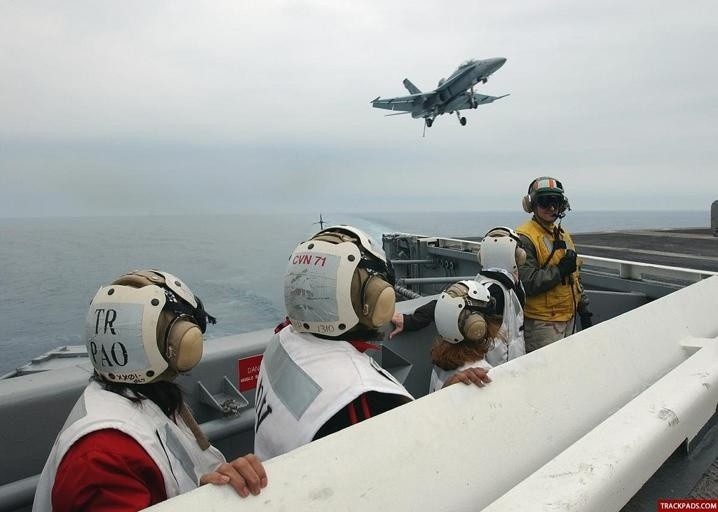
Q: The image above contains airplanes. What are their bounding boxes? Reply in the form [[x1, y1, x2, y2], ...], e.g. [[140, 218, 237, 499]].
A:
[[369, 57, 511, 138]]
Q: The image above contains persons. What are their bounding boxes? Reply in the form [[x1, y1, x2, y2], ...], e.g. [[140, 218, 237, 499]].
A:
[[28, 265, 268, 512], [251, 223, 491, 462], [428, 277, 494, 396], [515, 175, 591, 354], [389, 226, 526, 367]]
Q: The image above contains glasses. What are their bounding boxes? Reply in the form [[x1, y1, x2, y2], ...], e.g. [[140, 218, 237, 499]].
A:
[[533, 196, 564, 211]]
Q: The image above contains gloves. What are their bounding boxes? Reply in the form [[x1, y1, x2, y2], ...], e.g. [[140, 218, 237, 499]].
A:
[[577, 304, 593, 330], [557, 255, 578, 278]]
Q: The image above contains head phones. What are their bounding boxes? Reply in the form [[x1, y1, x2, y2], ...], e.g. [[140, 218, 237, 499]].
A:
[[163, 313, 204, 376], [361, 277, 397, 330], [463, 311, 487, 340], [522, 193, 571, 219], [477, 234, 526, 266]]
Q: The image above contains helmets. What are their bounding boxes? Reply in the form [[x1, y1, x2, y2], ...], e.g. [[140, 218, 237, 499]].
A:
[[83, 269, 216, 385], [284, 223, 396, 340], [435, 280, 492, 345], [523, 176, 571, 215], [478, 225, 527, 273]]
